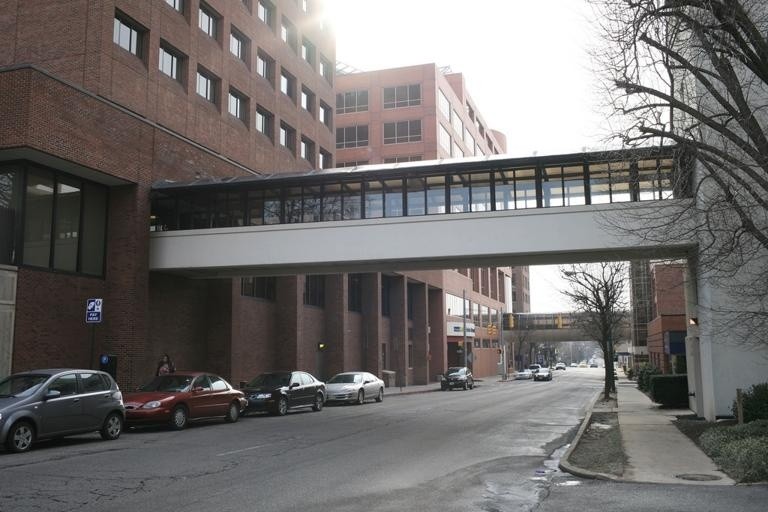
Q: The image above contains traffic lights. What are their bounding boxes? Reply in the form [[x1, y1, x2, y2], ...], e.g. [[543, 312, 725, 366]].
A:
[[491, 325, 496, 335], [496, 349, 501, 354], [487, 324, 491, 335], [457, 339, 464, 349]]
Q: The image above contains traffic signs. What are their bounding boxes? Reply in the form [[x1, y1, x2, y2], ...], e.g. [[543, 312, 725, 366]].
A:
[[85, 298, 102, 324]]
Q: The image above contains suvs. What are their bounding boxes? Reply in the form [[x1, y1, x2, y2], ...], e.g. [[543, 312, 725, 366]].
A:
[[555, 362, 566, 370]]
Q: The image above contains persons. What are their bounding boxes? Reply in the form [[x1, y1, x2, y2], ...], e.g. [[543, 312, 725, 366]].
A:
[[156, 354, 176, 387]]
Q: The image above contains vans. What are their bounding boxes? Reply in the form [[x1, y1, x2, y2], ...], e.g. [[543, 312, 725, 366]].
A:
[[528, 364, 541, 376]]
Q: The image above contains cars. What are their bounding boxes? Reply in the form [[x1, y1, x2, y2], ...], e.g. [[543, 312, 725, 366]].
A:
[[323, 372, 387, 404], [235, 368, 329, 416], [0, 368, 128, 452], [516, 369, 532, 380], [590, 362, 599, 368], [578, 362, 587, 368], [534, 367, 553, 380], [120, 371, 248, 430], [570, 362, 578, 367], [438, 366, 475, 390]]
